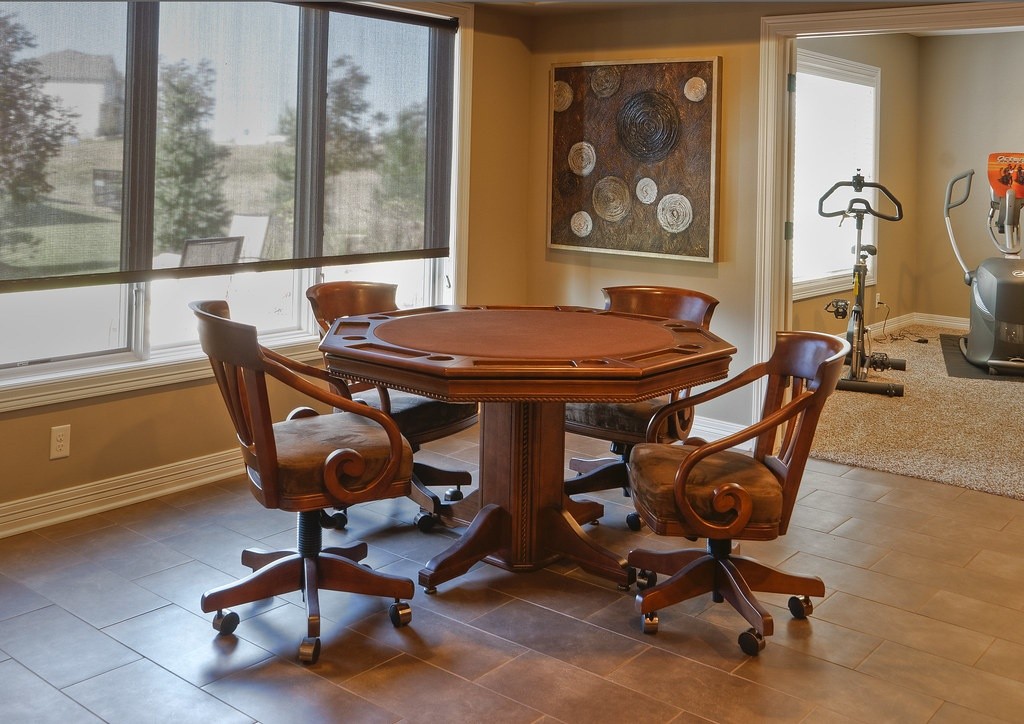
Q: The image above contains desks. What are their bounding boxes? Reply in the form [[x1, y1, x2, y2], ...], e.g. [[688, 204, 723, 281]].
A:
[[319, 303, 737, 595]]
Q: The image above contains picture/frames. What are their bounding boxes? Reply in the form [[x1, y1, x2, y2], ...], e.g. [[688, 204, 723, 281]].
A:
[[548, 56, 720, 265]]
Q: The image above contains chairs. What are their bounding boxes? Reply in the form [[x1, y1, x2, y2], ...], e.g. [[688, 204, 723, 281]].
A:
[[305, 280, 473, 530], [187, 299, 415, 665], [621, 330, 852, 657], [564, 284, 719, 534]]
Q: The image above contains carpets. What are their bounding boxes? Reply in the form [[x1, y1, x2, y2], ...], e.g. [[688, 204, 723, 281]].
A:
[[793, 323, 1024, 501]]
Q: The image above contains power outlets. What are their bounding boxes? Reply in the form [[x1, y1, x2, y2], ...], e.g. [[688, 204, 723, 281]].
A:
[[50, 425, 71, 460], [876, 293, 881, 309]]
